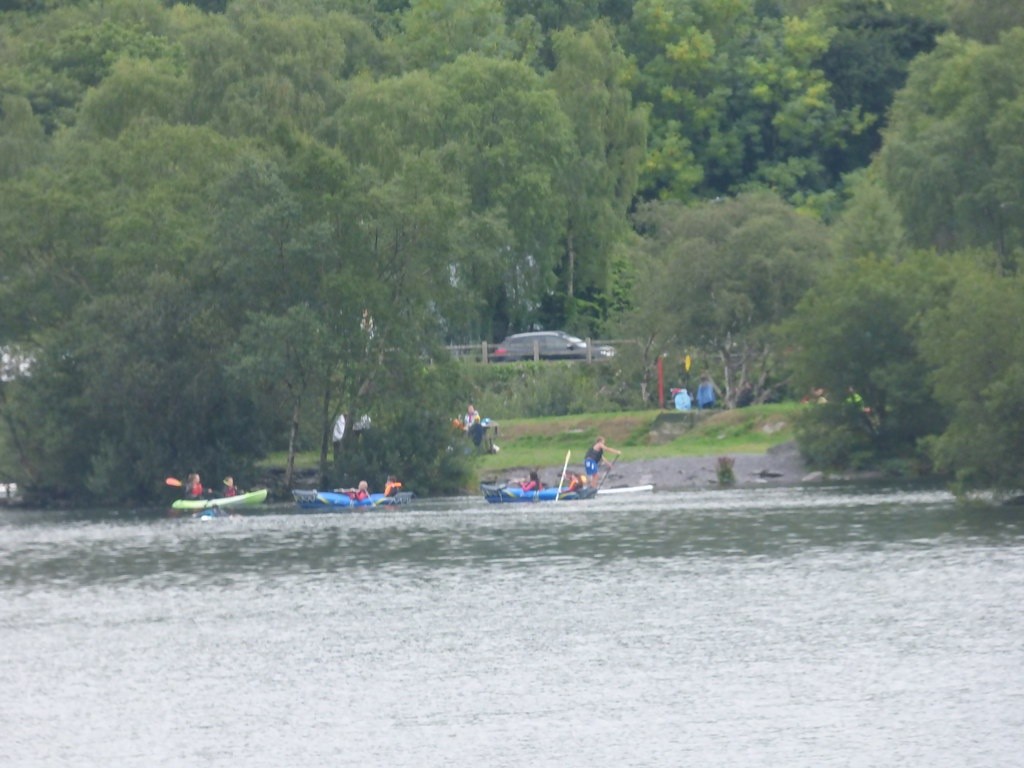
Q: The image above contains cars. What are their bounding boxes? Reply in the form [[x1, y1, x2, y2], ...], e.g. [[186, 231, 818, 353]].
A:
[[496, 330, 616, 362]]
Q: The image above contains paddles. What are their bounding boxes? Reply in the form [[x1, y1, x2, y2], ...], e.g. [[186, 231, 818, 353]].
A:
[[166, 477, 220, 495], [556, 449, 570, 500], [685, 355, 691, 391], [598, 453, 619, 490]]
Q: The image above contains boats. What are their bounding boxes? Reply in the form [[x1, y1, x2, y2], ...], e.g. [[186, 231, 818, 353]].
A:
[[479, 480, 596, 506], [169, 487, 269, 514], [291, 488, 413, 508]]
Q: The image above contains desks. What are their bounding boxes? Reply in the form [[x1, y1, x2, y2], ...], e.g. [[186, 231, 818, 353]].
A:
[[482, 422, 502, 454]]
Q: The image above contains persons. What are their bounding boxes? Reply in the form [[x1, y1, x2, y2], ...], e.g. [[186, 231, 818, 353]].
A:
[[224, 476, 235, 497], [384, 476, 398, 497], [188, 473, 212, 501], [350, 481, 369, 500], [559, 470, 583, 494], [520, 472, 542, 492], [584, 437, 621, 489], [672, 372, 864, 410], [464, 405, 483, 446]]
